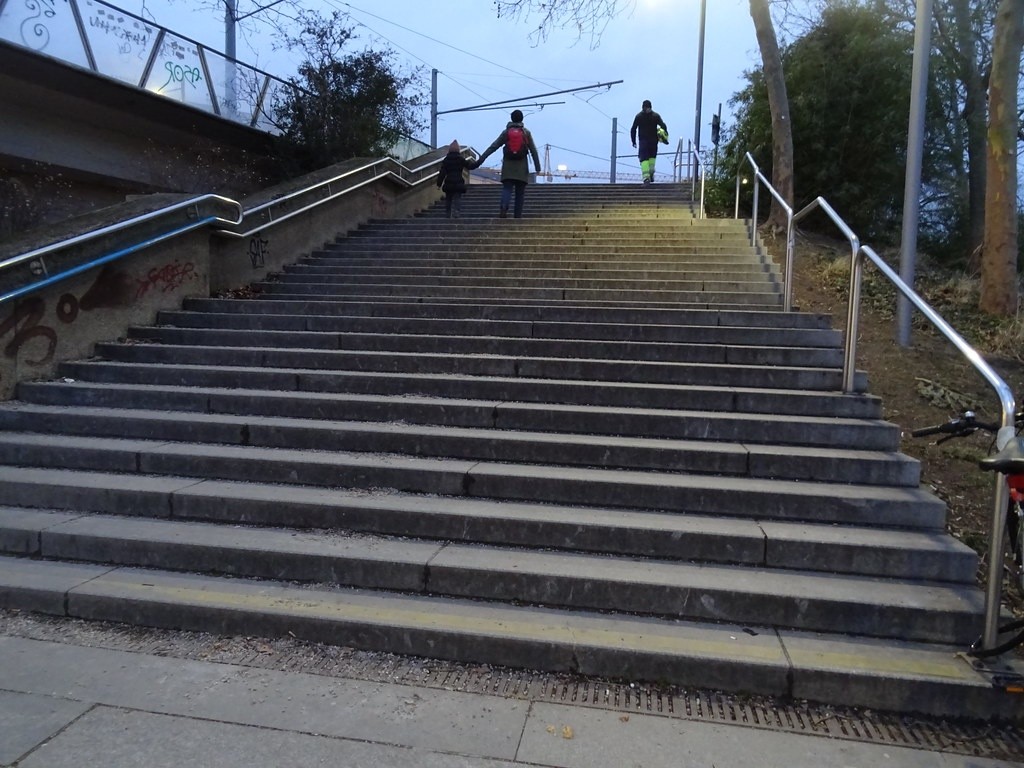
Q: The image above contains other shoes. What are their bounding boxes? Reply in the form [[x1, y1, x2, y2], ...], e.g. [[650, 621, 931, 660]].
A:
[[500, 208, 507, 218], [650, 175, 654, 182], [643, 178, 650, 189]]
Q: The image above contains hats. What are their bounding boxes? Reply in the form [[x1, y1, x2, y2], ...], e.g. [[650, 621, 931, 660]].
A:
[[449, 139, 460, 153]]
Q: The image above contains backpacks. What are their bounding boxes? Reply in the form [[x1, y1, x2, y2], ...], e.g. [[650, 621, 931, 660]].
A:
[[503, 126, 529, 160]]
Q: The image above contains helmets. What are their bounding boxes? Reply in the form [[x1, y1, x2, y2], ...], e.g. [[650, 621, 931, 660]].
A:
[[657, 127, 668, 142]]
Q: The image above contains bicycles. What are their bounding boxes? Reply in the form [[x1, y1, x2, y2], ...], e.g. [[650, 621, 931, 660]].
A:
[[911, 409, 1023, 598]]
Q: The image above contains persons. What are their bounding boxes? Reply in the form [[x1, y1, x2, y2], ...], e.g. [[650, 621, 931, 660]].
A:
[[630, 100, 668, 183], [476, 110, 541, 219], [436, 140, 484, 219]]
